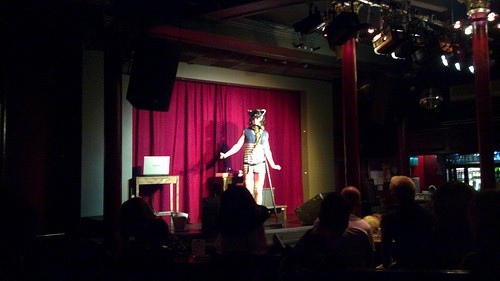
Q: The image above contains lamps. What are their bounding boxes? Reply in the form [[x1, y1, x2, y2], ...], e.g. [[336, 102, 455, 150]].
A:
[[292, 0, 500, 110]]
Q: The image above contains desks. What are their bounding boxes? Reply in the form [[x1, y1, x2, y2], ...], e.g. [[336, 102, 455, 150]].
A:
[[135, 176, 180, 231]]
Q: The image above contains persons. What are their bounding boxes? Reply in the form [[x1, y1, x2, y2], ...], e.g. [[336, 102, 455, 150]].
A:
[[118, 176, 500, 281], [220, 109, 281, 206]]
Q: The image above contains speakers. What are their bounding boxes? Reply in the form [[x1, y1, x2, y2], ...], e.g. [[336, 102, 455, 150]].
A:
[[126, 35, 182, 112]]
